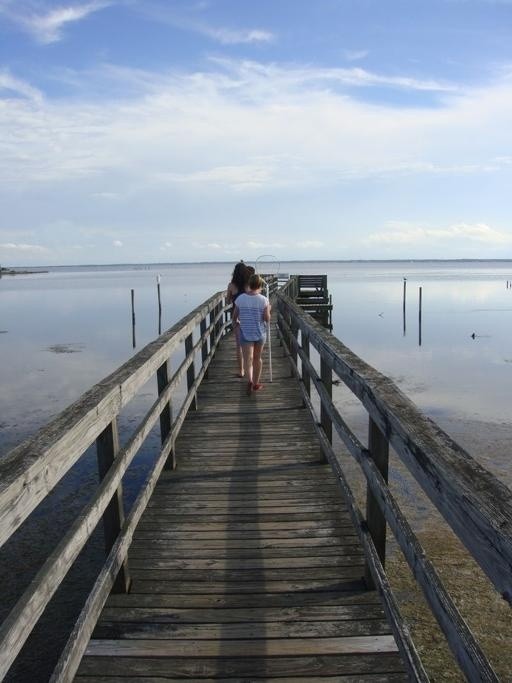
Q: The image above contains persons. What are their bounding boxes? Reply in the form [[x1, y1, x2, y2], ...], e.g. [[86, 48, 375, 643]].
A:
[[226, 259, 272, 392]]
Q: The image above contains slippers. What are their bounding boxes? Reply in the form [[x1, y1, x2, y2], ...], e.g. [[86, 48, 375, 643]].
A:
[[239, 371, 246, 378]]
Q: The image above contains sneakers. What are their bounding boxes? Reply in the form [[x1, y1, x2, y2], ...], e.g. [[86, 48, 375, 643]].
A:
[[253, 384, 265, 390], [247, 382, 254, 397]]
